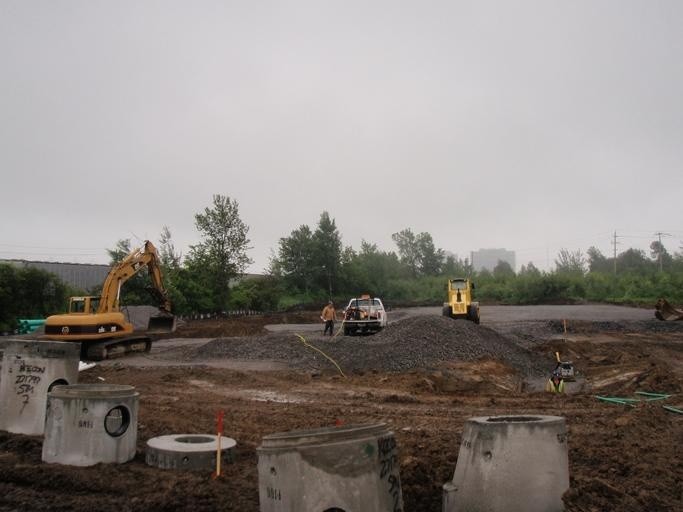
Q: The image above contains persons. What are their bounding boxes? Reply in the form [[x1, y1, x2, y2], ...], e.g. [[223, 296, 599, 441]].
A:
[[321, 300, 338, 337]]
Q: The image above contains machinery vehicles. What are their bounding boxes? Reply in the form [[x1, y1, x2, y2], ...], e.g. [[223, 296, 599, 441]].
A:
[[35, 240, 177, 362], [443, 278, 480, 324]]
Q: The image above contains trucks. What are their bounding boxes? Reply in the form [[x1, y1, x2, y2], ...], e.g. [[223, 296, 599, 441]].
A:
[[342, 295, 389, 337]]
